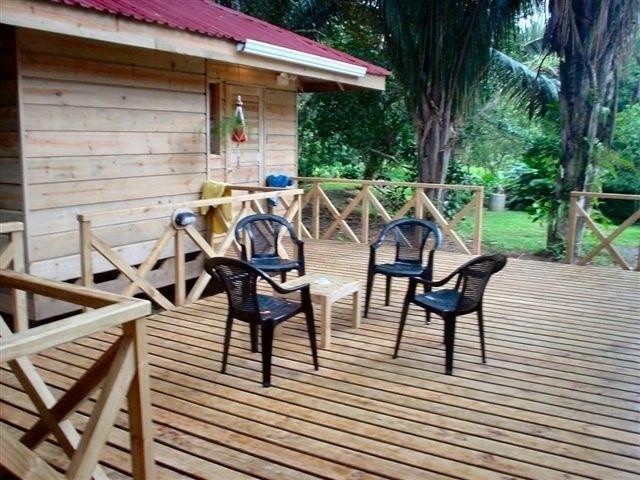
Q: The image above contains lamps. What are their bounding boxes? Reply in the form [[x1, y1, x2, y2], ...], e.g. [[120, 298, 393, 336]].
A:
[[275, 69, 300, 91]]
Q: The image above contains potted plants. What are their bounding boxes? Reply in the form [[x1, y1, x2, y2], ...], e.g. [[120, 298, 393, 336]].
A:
[[213, 115, 249, 146]]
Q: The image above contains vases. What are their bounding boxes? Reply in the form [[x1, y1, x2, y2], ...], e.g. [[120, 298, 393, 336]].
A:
[[488, 194, 507, 211]]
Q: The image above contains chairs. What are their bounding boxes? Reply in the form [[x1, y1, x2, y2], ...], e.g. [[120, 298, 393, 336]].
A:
[[232, 214, 309, 284], [361, 216, 444, 324], [199, 256, 324, 390], [391, 248, 509, 379]]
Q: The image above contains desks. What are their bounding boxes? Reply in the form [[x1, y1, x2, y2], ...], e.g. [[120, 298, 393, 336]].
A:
[[268, 266, 362, 349]]
